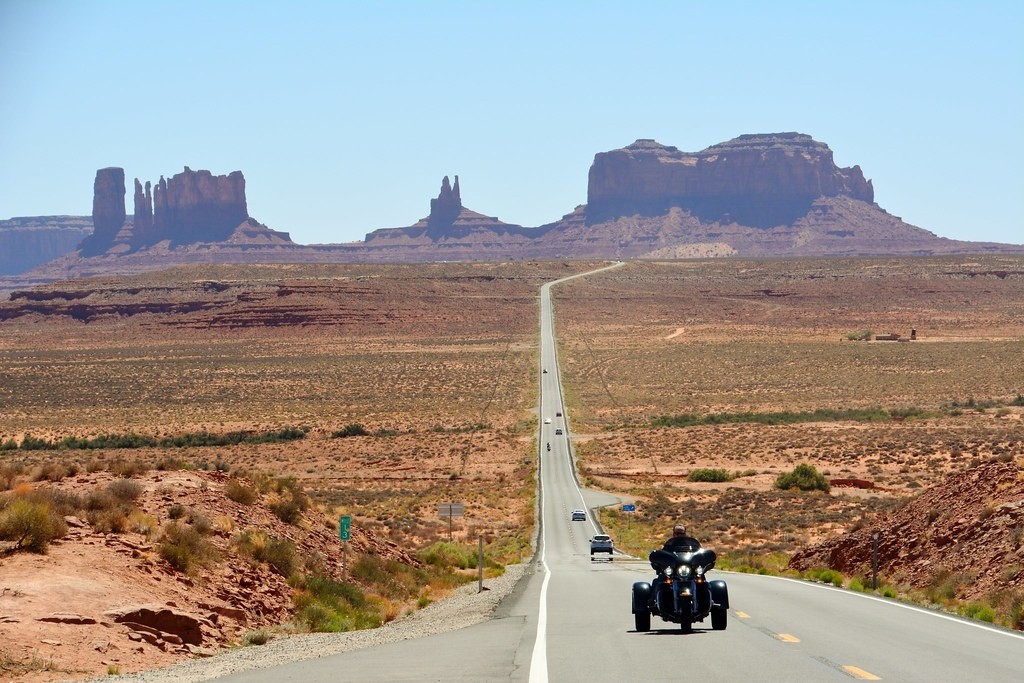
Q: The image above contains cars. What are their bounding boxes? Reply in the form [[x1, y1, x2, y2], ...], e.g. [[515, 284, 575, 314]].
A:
[[572, 509, 586, 521], [556, 413, 561, 416], [556, 428, 562, 435], [543, 369, 547, 373], [544, 418, 551, 424]]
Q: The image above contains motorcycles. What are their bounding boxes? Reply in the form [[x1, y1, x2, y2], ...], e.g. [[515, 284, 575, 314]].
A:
[[547, 445, 551, 451], [630, 535, 729, 634]]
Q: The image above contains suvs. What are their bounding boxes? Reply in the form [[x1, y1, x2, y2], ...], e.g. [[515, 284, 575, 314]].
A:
[[589, 535, 614, 554]]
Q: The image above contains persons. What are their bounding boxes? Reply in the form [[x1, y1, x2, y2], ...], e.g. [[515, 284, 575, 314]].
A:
[[663, 525, 701, 552], [547, 442, 550, 448]]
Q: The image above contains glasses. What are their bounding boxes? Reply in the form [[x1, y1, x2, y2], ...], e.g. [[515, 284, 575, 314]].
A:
[[673, 531, 685, 535]]
